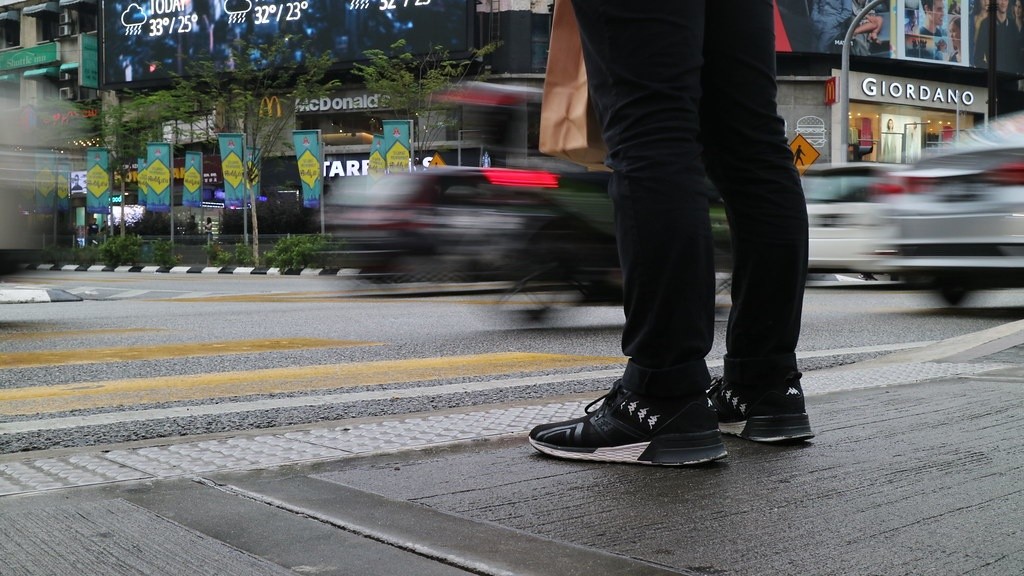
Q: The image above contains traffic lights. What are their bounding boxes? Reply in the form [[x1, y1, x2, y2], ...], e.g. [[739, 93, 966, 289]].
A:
[[853, 142, 873, 162]]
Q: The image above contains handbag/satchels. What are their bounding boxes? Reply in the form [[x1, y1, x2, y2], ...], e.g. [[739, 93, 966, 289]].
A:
[[540, 0, 613, 173]]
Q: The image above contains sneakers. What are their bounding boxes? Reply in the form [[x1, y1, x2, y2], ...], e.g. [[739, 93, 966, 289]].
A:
[[528, 379, 727, 465], [706, 372, 815, 442]]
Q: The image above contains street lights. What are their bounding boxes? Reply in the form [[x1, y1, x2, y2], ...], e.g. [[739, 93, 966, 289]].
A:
[[457, 126, 494, 166]]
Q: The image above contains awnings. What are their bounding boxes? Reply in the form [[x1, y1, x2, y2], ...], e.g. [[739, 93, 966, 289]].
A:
[[23, 1, 64, 17], [0, 74, 20, 83], [0, 10, 20, 21], [23, 61, 79, 79], [59, 0, 98, 12]]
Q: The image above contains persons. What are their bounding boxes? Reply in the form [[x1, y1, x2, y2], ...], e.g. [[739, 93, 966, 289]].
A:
[[527, 0, 815, 465], [184, 215, 199, 244], [904, 0, 962, 63], [910, 121, 919, 162], [968, 0, 1024, 75], [205, 217, 212, 235], [72, 174, 82, 190], [87, 218, 110, 247], [810, 0, 883, 52], [883, 119, 896, 163]]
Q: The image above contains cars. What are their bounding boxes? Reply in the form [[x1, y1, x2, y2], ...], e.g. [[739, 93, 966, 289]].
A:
[[800, 161, 906, 275], [877, 108, 1024, 307], [314, 165, 549, 283], [447, 158, 739, 319]]
